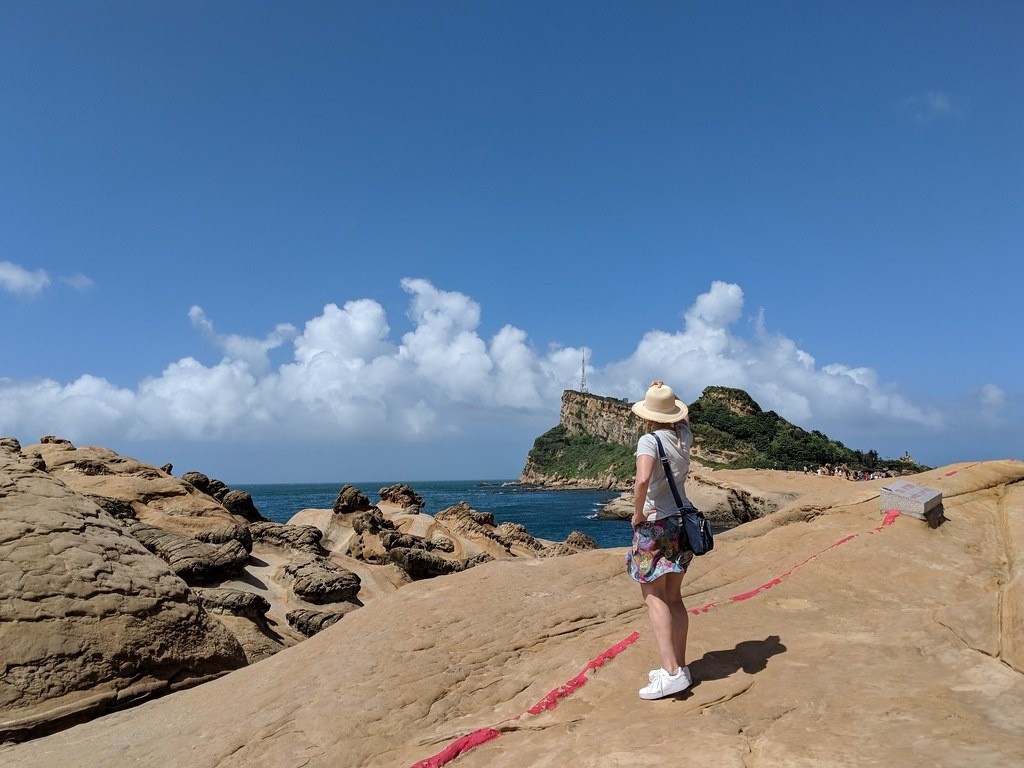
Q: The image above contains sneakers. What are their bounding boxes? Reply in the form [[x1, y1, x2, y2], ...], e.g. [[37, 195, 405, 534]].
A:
[[639, 666, 692, 700]]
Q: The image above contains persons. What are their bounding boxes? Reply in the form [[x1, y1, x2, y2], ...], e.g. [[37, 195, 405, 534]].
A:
[[803, 462, 895, 483], [624, 379, 694, 700]]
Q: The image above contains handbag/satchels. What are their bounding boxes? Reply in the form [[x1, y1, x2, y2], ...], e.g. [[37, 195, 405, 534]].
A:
[[680, 508, 715, 556]]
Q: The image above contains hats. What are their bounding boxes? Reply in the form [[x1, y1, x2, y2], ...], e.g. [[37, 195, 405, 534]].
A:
[[631, 384, 688, 423]]
